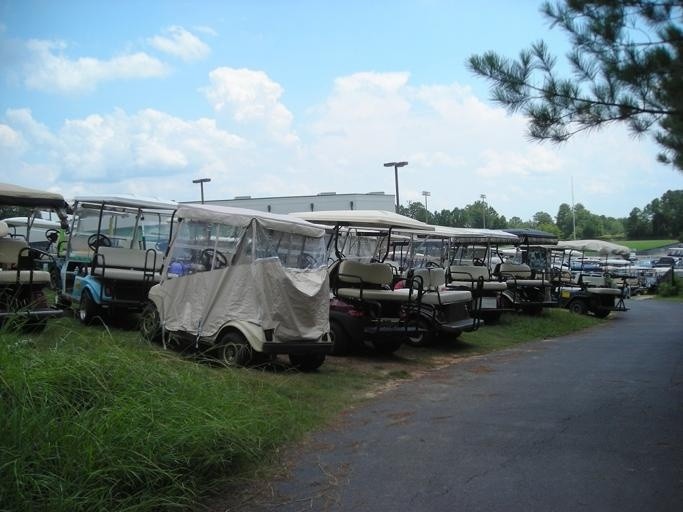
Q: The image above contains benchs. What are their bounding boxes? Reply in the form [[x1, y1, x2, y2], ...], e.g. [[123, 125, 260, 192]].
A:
[[498, 260, 550, 290], [447, 263, 506, 293], [414, 266, 473, 308], [338, 259, 417, 305], [93, 243, 165, 286], [1, 235, 50, 283], [583, 271, 622, 298]]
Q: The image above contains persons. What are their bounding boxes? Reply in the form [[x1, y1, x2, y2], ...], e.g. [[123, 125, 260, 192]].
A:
[[622, 279, 630, 299], [637, 272, 646, 286]]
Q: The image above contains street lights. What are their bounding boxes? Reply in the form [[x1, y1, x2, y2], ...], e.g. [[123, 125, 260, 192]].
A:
[[193, 177, 212, 203], [384, 160, 407, 214], [422, 190, 430, 224], [481, 194, 486, 228]]
